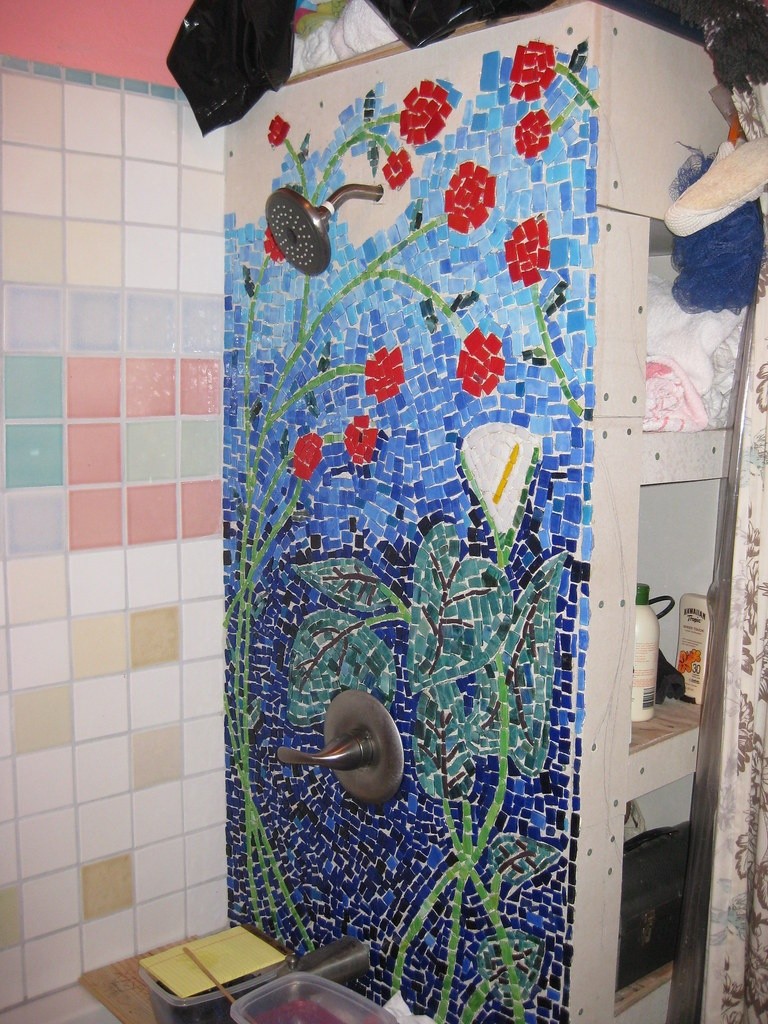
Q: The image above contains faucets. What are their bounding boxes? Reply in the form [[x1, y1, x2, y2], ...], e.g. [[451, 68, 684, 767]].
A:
[[286, 938, 372, 1000]]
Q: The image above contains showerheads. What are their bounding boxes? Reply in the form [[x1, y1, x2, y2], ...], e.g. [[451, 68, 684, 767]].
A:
[[265, 185, 332, 277]]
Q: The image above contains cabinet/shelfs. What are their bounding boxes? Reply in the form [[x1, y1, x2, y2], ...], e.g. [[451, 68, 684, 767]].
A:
[[565, 0, 768, 1024]]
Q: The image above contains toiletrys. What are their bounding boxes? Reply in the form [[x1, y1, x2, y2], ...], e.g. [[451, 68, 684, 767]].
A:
[[632, 582, 660, 723], [678, 588, 712, 701]]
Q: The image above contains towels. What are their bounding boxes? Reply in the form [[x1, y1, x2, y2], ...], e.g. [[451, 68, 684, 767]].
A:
[[284, 0, 399, 79], [645, 270, 747, 432]]
